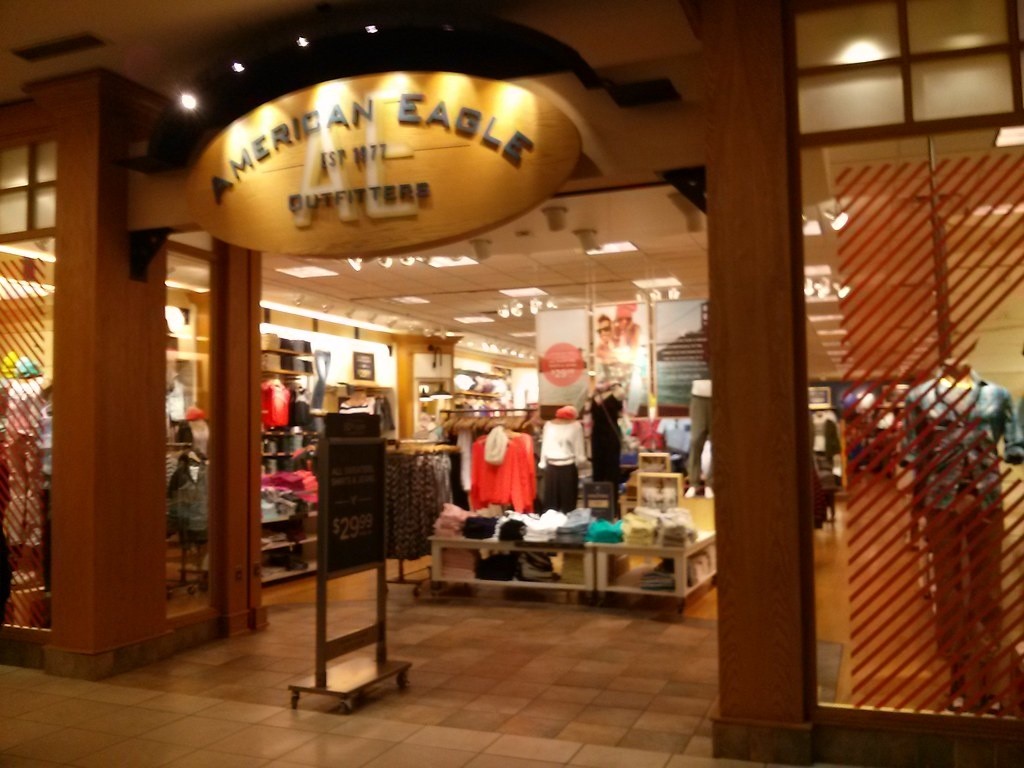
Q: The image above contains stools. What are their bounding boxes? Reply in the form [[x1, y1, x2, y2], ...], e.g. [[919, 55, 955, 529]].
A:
[[582, 481, 614, 522]]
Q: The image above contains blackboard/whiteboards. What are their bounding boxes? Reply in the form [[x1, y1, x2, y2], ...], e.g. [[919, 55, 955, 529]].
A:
[[318, 438, 386, 580]]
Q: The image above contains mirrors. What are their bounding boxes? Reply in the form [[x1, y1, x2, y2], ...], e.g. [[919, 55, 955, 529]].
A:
[[417, 381, 446, 431]]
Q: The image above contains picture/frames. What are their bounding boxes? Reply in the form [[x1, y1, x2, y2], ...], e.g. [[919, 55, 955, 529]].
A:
[[808, 386, 830, 410], [882, 384, 908, 407]]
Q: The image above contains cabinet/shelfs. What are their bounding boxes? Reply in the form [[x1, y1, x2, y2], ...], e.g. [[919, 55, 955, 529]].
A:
[[594, 529, 718, 615], [426, 532, 595, 599], [164, 332, 392, 583]]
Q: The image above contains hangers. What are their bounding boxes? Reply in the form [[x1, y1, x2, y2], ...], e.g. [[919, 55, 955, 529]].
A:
[[167, 374, 540, 467]]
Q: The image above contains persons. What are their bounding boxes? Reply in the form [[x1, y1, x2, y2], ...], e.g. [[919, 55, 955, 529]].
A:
[[656, 417, 691, 476], [339, 387, 376, 415], [687, 379, 716, 496], [32, 404, 53, 629], [631, 416, 689, 475], [587, 384, 627, 484], [810, 411, 843, 522], [174, 409, 210, 460], [901, 357, 1024, 714], [594, 314, 648, 395], [537, 418, 586, 515], [313, 350, 331, 408]]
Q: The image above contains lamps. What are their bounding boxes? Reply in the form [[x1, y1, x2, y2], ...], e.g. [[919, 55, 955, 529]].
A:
[[576, 229, 604, 256], [544, 207, 566, 232], [472, 241, 493, 263], [418, 387, 432, 401], [683, 205, 704, 235], [429, 380, 452, 400]]
